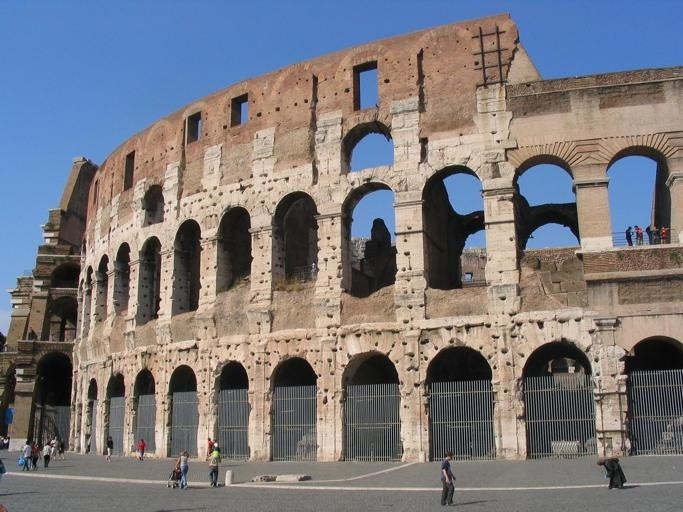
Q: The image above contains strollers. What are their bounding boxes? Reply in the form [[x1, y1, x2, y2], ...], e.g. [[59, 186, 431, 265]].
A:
[[167, 464, 181, 488]]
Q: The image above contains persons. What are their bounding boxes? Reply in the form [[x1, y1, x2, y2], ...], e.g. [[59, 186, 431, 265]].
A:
[[594, 458, 628, 490], [137, 438, 147, 461], [0, 432, 9, 450], [86, 435, 90, 452], [212, 438, 220, 460], [175, 449, 190, 491], [104, 436, 114, 462], [206, 437, 213, 454], [16, 435, 65, 474], [625, 224, 669, 246], [440, 451, 457, 506], [207, 447, 222, 488], [310, 260, 316, 279]]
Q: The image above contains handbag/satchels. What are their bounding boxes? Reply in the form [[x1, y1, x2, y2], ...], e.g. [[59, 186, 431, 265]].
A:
[[15, 455, 26, 466]]
